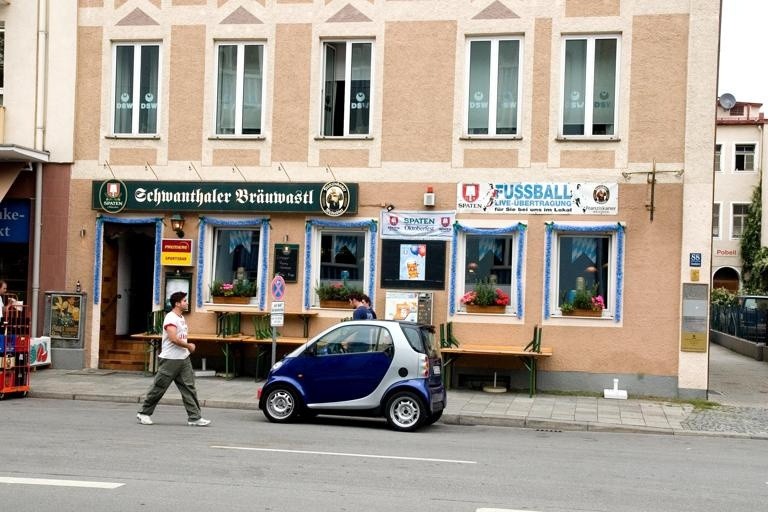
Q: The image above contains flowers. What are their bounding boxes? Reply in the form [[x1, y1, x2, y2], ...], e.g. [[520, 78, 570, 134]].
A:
[[209, 278, 256, 298], [560, 280, 605, 312], [460, 279, 509, 306], [316, 285, 363, 302]]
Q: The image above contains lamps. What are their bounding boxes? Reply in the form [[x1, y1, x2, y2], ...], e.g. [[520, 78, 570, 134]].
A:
[[169, 212, 185, 238]]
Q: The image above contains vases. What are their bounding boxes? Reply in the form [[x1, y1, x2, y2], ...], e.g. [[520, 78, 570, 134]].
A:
[[212, 295, 251, 304], [562, 309, 602, 317], [466, 303, 506, 314], [320, 300, 358, 309]]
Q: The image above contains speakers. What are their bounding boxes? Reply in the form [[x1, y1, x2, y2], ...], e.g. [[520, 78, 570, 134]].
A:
[[424, 192, 435, 206]]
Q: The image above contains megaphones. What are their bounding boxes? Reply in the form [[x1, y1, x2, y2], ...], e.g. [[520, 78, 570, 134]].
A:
[[719, 93, 737, 109]]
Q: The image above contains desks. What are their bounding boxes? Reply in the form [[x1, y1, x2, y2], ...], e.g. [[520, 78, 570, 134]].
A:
[[431, 321, 552, 397], [129, 307, 318, 383]]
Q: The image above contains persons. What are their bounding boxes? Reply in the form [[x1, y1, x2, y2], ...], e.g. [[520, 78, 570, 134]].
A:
[[346, 291, 373, 353], [0, 280, 13, 334], [361, 294, 378, 350], [136, 290, 212, 426]]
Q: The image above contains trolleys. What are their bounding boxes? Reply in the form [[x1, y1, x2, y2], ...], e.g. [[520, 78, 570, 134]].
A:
[[0, 293, 31, 400]]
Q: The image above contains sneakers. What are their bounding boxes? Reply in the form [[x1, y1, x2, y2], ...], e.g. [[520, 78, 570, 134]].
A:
[[136, 413, 153, 425], [188, 418, 211, 426]]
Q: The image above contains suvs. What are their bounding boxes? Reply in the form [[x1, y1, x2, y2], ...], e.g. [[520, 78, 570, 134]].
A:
[[718, 295, 768, 341]]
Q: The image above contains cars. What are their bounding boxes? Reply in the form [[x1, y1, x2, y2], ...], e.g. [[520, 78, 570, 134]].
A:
[[256, 317, 449, 432]]
